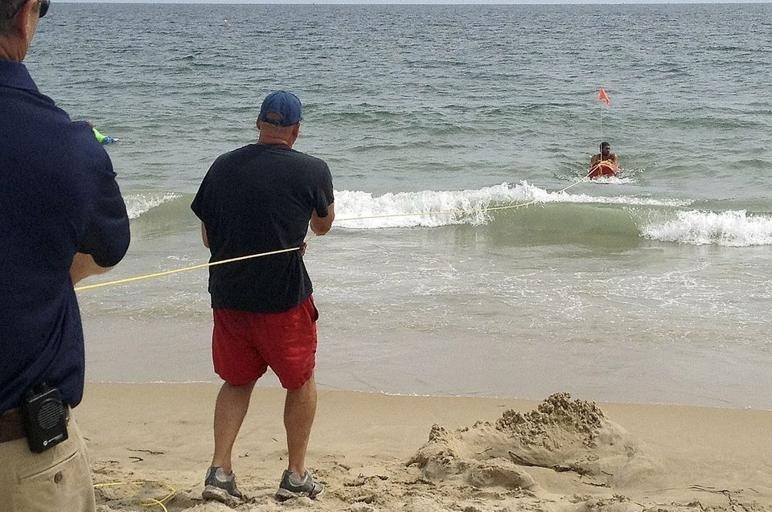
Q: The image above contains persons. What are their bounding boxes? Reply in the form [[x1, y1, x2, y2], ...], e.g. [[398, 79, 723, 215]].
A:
[[191, 89, 334, 505], [1, 0, 132, 512], [591, 142, 618, 169]]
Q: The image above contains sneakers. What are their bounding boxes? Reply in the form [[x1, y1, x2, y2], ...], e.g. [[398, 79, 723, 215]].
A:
[[276, 471, 325, 498], [201, 467, 242, 502]]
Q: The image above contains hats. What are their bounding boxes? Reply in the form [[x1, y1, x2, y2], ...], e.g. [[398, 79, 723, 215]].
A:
[[258, 92, 302, 125]]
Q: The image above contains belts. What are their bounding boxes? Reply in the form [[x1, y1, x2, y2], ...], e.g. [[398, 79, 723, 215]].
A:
[[0, 410, 28, 442]]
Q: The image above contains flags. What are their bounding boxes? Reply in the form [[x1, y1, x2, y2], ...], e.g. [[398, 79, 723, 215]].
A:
[[599, 89, 610, 105]]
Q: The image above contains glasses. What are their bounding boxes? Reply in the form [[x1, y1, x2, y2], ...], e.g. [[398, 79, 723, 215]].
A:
[[37, 0, 50, 18]]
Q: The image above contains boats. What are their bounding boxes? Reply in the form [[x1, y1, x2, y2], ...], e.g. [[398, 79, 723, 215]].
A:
[[587, 162, 622, 181]]
